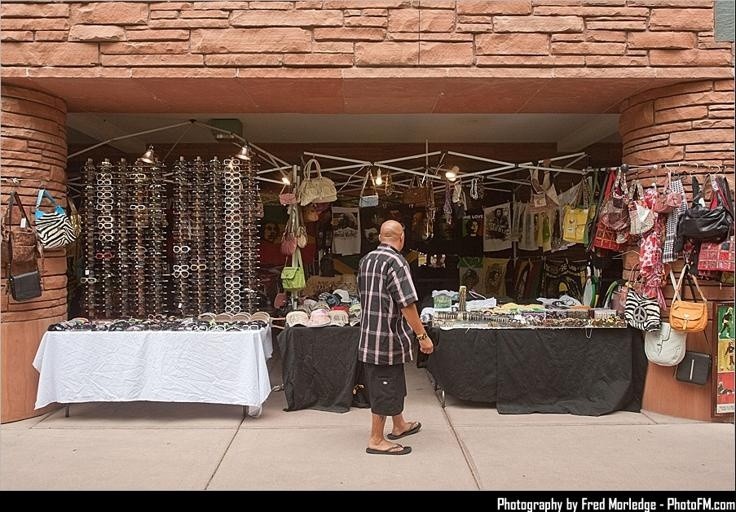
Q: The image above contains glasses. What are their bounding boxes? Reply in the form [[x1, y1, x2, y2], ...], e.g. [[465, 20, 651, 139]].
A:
[[81, 159, 257, 319], [47, 317, 267, 331]]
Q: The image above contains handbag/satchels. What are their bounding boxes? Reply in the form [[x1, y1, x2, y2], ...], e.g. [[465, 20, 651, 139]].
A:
[[32, 190, 81, 252], [273, 158, 338, 309], [623, 173, 736, 386], [0, 192, 42, 301], [359, 195, 379, 208], [403, 175, 429, 208], [565, 197, 628, 253]]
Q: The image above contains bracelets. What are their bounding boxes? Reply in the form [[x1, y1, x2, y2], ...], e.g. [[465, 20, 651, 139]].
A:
[[416, 333, 427, 340]]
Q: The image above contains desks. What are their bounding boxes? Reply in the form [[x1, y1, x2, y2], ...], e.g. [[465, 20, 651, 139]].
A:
[[278, 325, 369, 413], [39, 319, 271, 418], [418, 324, 646, 411]]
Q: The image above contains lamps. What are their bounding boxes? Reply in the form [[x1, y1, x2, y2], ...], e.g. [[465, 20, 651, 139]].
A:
[[138, 143, 157, 165], [235, 141, 252, 161], [209, 117, 243, 142], [281, 171, 293, 186], [445, 165, 460, 182]]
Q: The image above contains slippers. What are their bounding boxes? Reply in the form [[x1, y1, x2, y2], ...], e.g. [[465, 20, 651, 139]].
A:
[[365, 443, 412, 455], [387, 422, 422, 440]]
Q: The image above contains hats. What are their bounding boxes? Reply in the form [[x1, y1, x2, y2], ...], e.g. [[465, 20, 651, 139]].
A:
[[285, 289, 365, 328]]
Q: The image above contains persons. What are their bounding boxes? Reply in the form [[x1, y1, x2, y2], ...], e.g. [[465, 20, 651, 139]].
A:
[[356, 219, 434, 455], [488, 272, 500, 296], [408, 208, 514, 240], [261, 218, 278, 243]]
